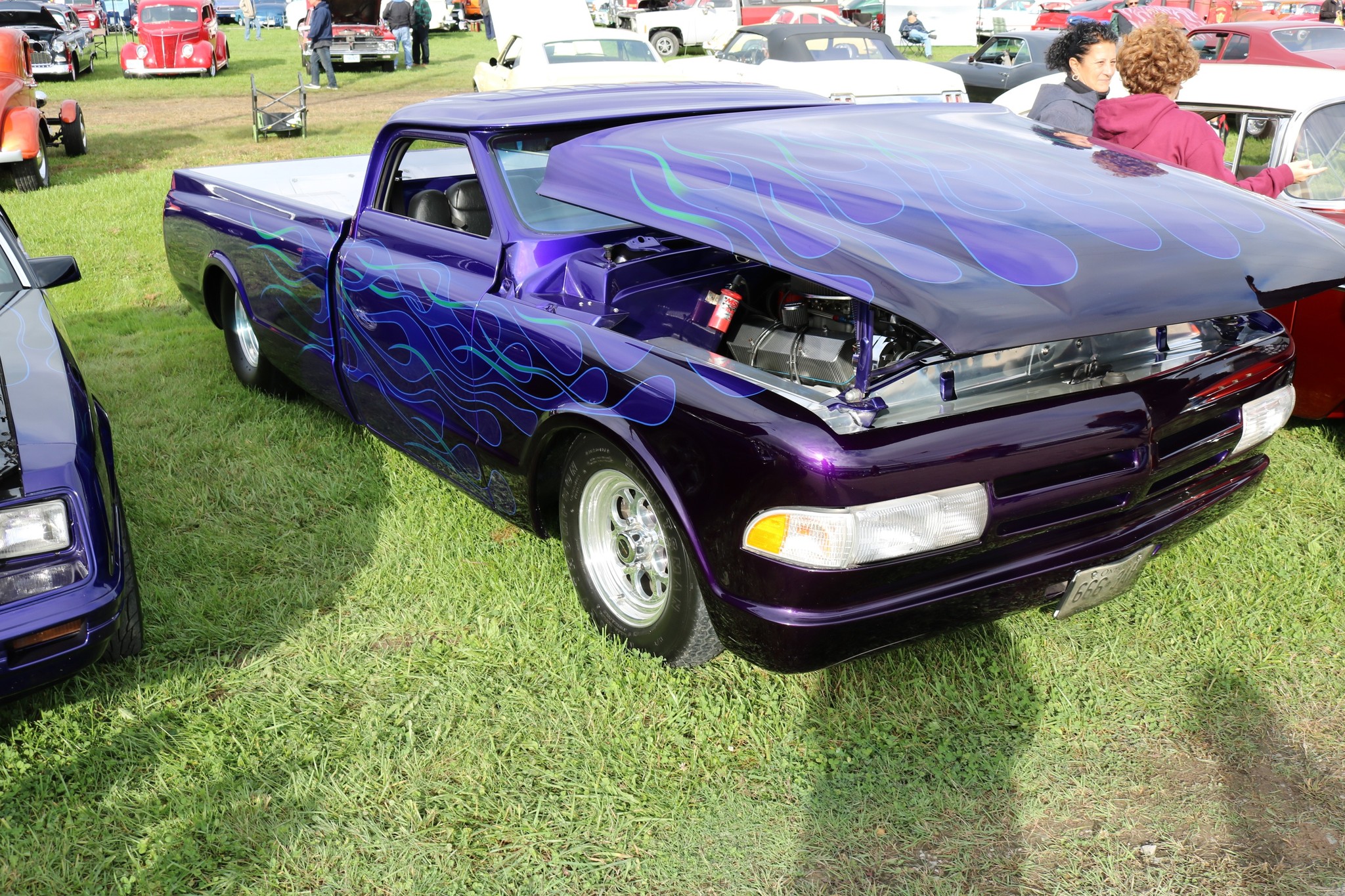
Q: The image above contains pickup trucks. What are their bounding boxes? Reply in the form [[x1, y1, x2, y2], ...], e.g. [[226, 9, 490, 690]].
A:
[[160, 91, 1345, 680], [593, 0, 842, 59]]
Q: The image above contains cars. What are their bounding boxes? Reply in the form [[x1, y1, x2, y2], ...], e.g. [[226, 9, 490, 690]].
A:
[[1183, 19, 1345, 72], [969, 0, 1345, 62], [986, 62, 1345, 231], [472, 26, 665, 94], [924, 29, 1120, 104], [210, 0, 285, 30], [0, 204, 145, 705], [297, 0, 399, 73], [665, 22, 972, 107]]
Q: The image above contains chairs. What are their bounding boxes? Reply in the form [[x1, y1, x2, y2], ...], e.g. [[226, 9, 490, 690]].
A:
[[852, 12, 873, 30], [900, 31, 926, 57], [249, 72, 309, 142], [993, 17, 1016, 34], [408, 175, 548, 236]]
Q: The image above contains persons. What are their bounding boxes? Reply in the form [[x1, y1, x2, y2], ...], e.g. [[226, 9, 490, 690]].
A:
[[1090, 13, 1330, 199], [902, 10, 937, 59], [411, 0, 432, 66], [1028, 0, 1146, 140], [129, 0, 138, 16], [382, 0, 416, 70], [701, 2, 719, 56], [239, 0, 263, 42], [1001, 50, 1012, 67], [307, 0, 339, 91], [1319, 0, 1345, 25], [479, 0, 497, 42]]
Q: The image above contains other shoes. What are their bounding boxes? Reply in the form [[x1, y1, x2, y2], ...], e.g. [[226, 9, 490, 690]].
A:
[[929, 35, 937, 39], [304, 83, 321, 89], [419, 63, 427, 67], [492, 37, 496, 41], [406, 65, 411, 69], [412, 63, 420, 66], [327, 85, 338, 90], [394, 66, 397, 69], [256, 37, 264, 40], [926, 55, 933, 59]]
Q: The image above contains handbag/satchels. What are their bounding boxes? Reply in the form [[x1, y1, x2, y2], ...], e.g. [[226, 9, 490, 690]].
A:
[[1333, 11, 1343, 26]]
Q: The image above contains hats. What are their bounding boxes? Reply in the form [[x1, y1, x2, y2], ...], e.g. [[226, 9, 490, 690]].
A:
[[907, 10, 918, 16]]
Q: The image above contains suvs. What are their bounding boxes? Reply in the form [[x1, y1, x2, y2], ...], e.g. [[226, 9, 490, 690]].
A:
[[120, 0, 231, 79], [0, 0, 109, 191]]
